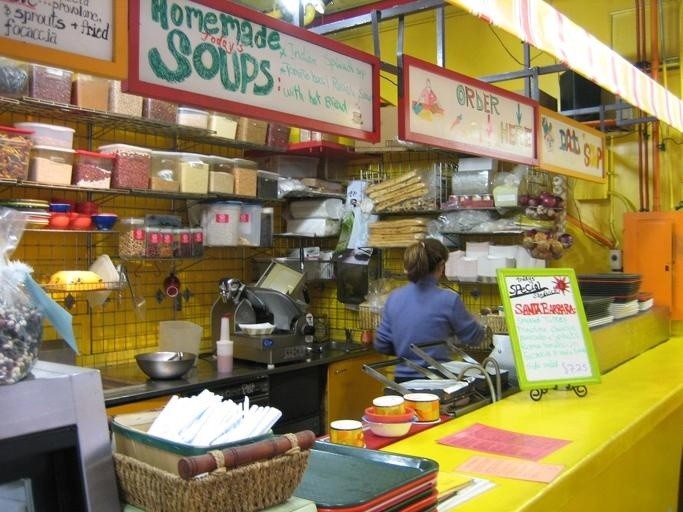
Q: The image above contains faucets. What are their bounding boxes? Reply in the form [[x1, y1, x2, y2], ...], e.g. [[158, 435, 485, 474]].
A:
[[455, 363, 495, 403], [481, 356, 502, 402]]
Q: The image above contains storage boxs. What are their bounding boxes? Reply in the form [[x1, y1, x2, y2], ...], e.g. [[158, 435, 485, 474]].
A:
[[0, 57, 126, 98]]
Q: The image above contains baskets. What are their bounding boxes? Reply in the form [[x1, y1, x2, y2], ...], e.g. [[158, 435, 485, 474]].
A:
[[450, 331, 492, 353], [111, 452, 310, 512], [486, 314, 509, 335]]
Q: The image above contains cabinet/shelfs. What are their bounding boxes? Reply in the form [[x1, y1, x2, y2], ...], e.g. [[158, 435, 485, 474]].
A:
[[0, 96, 682, 511]]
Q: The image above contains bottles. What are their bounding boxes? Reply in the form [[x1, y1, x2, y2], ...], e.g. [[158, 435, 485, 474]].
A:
[[216, 317, 234, 373]]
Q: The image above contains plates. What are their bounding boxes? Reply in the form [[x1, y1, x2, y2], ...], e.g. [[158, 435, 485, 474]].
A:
[[0, 197, 50, 229], [410, 418, 440, 425], [441, 238, 550, 287]]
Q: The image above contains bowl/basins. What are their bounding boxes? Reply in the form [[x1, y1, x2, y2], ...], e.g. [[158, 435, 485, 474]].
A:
[[133, 351, 195, 379], [46, 200, 118, 231], [362, 393, 416, 438]]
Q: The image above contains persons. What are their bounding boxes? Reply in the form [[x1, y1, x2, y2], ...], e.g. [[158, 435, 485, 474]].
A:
[[372, 238, 484, 390]]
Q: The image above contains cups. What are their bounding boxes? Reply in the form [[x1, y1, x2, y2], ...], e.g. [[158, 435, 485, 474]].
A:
[[403, 393, 440, 422], [329, 420, 364, 449]]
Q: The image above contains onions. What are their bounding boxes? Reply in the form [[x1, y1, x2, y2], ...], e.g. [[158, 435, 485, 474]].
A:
[[519, 191, 565, 208]]
